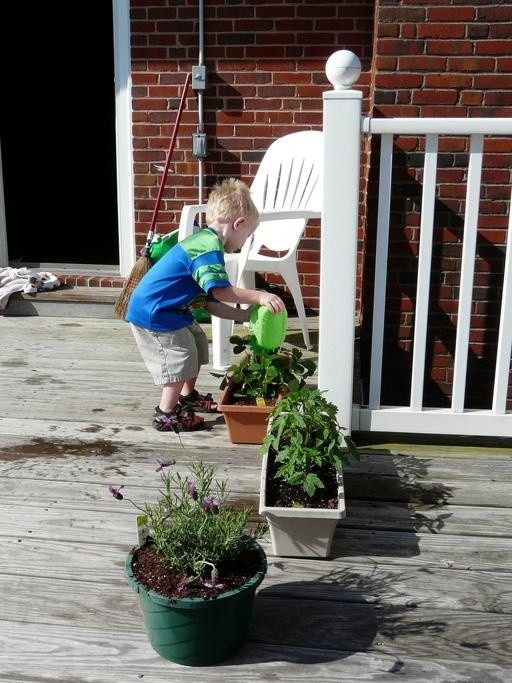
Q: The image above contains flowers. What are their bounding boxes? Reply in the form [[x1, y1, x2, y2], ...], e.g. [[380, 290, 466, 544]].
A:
[[107, 407, 272, 593]]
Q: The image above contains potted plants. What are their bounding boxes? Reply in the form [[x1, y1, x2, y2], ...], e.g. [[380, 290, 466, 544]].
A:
[[255, 377, 362, 560], [208, 332, 314, 448]]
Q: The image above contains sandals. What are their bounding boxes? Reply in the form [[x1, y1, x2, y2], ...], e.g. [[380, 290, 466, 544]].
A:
[[176, 390, 218, 411], [153, 405, 206, 431]]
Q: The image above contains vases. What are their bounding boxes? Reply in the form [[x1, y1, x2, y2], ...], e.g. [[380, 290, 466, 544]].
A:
[[123, 538, 269, 666]]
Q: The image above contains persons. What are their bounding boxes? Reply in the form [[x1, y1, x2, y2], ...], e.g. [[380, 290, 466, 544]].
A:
[[124, 177, 288, 433]]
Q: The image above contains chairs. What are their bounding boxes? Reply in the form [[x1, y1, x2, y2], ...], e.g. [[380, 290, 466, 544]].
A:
[[173, 128, 323, 374]]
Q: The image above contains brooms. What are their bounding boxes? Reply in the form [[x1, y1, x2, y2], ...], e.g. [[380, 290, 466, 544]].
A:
[[114, 71, 193, 321]]
